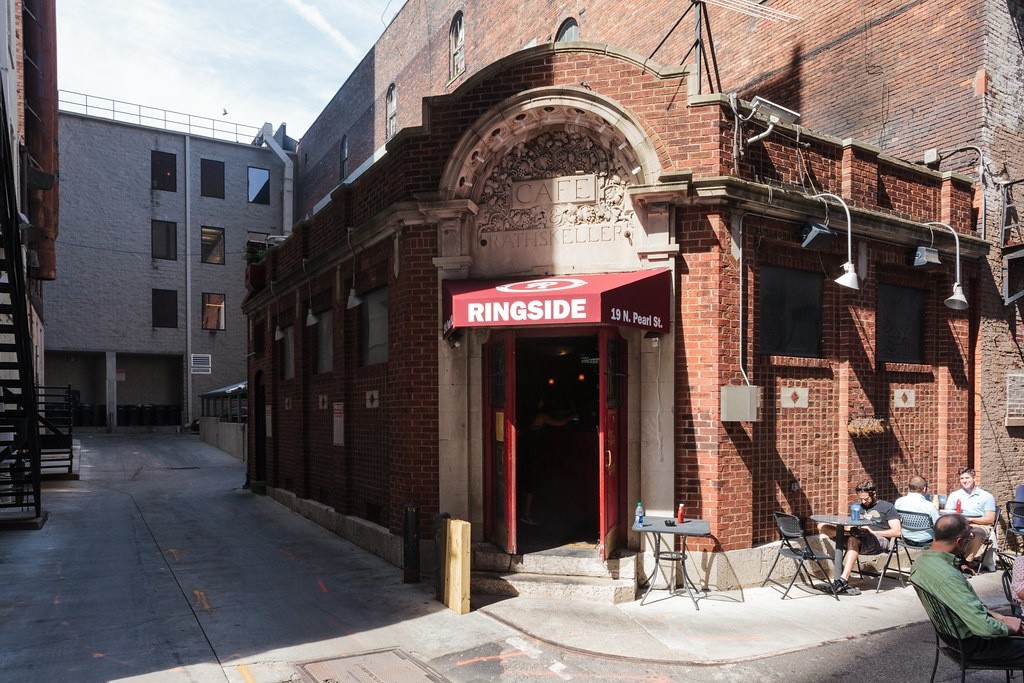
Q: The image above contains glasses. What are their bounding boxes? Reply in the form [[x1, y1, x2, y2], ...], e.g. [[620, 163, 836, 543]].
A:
[[960, 531, 975, 539]]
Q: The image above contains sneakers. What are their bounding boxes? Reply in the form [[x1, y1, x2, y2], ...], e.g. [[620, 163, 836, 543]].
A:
[[849, 527, 876, 548], [827, 577, 848, 594]]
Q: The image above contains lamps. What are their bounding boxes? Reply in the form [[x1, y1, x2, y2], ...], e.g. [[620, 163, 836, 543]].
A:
[[346, 226, 365, 311], [448, 332, 464, 349], [923, 221, 969, 311], [303, 258, 321, 327], [275, 296, 288, 341], [644, 331, 664, 347], [812, 194, 861, 292], [801, 196, 837, 249], [923, 148, 941, 165], [743, 96, 801, 147], [913, 224, 941, 269]]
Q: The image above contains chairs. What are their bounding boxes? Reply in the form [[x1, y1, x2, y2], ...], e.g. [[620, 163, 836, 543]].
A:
[[1001, 500, 1024, 562], [910, 580, 1024, 683], [951, 504, 1002, 576], [762, 510, 841, 602], [1002, 568, 1024, 679], [830, 505, 935, 594]]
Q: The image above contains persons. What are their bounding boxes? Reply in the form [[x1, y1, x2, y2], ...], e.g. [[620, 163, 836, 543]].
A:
[[908, 514, 1024, 669], [817, 478, 902, 595], [894, 475, 941, 547], [1009, 551, 1024, 624], [944, 467, 996, 573]]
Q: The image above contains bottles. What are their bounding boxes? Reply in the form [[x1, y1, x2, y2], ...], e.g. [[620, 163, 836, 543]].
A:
[[678, 504, 685, 524], [635, 502, 644, 528], [850, 503, 861, 522], [956, 498, 961, 513], [938, 495, 946, 510]]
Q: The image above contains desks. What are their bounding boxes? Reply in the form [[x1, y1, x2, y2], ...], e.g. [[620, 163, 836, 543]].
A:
[[633, 515, 712, 611], [939, 508, 983, 579], [810, 514, 876, 597]]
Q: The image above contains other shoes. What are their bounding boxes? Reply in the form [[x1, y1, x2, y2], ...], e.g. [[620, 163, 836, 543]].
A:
[[521, 517, 536, 525]]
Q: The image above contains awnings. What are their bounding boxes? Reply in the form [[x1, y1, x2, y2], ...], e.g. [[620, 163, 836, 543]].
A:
[[441, 267, 672, 340]]
